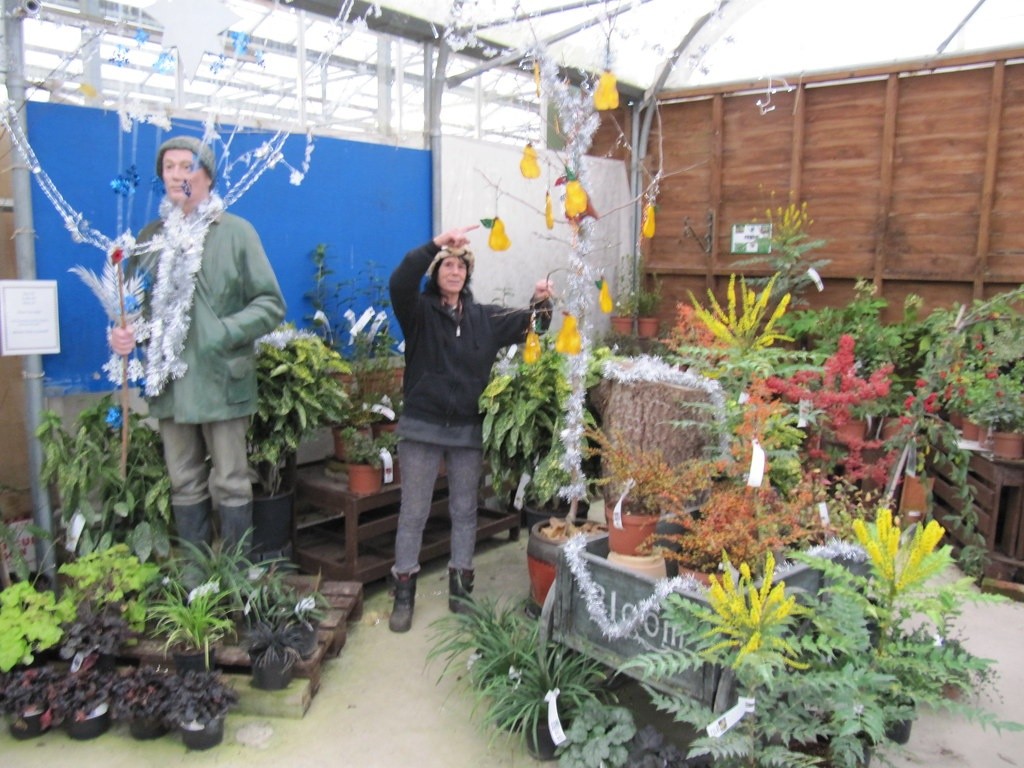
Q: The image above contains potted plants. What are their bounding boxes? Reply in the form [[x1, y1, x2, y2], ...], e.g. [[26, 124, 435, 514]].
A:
[[0, 186, 1024, 768]]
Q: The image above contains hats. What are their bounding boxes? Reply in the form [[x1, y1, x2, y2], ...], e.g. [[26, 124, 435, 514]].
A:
[[426, 245, 475, 280]]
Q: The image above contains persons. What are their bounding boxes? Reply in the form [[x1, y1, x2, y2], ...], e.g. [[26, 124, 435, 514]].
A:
[[388, 224, 558, 632], [112, 136, 286, 591]]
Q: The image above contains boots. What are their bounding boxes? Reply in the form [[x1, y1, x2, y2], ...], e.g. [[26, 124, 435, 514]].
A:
[[390, 563, 420, 632], [448, 560, 482, 628]]
